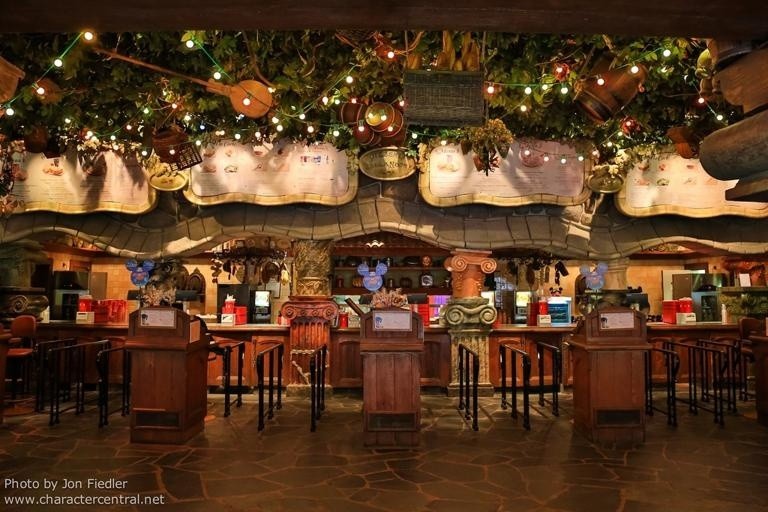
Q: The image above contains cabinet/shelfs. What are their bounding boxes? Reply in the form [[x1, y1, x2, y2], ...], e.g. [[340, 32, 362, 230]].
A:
[[334, 265, 449, 294]]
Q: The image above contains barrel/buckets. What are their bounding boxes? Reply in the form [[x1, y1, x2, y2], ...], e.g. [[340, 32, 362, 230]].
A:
[[339, 97, 407, 149], [548, 295, 573, 327], [574, 50, 649, 126]]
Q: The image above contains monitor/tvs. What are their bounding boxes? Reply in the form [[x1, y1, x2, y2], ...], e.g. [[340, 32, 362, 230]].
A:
[[250, 290, 272, 314]]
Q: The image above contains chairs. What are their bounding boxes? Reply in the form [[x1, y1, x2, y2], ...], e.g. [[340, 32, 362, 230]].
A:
[[6, 315, 37, 399]]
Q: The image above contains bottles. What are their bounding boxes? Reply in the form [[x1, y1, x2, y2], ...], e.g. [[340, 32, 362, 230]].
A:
[[721, 304, 728, 325]]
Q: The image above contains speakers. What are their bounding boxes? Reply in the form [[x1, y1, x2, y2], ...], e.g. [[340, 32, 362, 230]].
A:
[[402, 70, 485, 127]]
